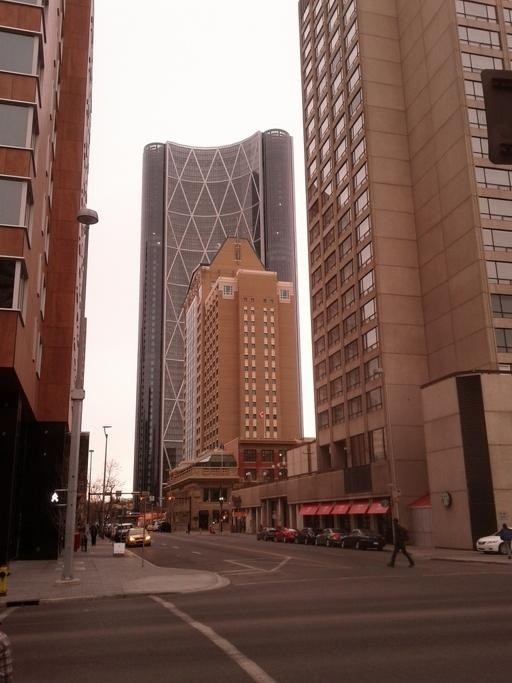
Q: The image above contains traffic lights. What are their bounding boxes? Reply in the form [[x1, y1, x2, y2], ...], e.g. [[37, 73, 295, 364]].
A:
[[49, 489, 67, 506]]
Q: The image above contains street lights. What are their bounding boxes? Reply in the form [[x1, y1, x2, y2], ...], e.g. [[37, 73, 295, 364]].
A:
[[99, 426, 112, 537], [371, 366, 402, 544], [62, 206, 99, 576], [216, 437, 226, 533], [87, 450, 94, 524]]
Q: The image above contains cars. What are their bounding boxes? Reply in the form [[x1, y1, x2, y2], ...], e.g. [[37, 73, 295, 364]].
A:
[[104, 520, 171, 547], [476, 528, 512, 555], [256, 525, 387, 550]]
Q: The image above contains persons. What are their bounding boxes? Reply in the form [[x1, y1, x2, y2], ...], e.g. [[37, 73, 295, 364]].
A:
[[500, 522, 512, 558], [76, 520, 88, 552], [88, 521, 98, 546], [386, 517, 415, 568]]
